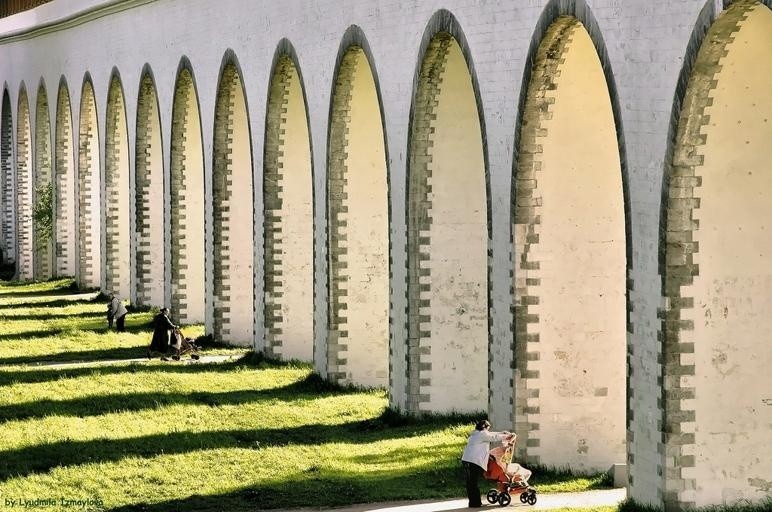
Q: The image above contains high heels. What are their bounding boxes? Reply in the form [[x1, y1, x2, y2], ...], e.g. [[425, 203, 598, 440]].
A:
[[146, 352, 170, 361]]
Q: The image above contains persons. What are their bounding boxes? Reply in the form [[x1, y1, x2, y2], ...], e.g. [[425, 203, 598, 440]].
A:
[[107, 292, 128, 332], [146, 307, 180, 361], [462, 419, 517, 507]]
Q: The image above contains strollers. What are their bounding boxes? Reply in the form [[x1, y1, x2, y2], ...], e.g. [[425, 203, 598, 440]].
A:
[[483, 433, 537, 506], [172, 324, 202, 361]]
